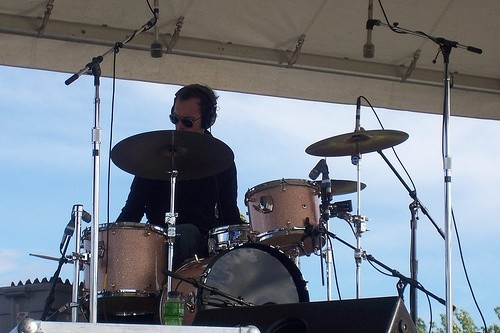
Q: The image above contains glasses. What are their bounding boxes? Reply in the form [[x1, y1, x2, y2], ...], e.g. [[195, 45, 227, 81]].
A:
[[169, 110, 207, 126]]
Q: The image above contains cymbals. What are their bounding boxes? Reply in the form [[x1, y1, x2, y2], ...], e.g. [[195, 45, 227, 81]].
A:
[[305, 130, 409, 157], [110, 130, 235, 179], [313, 179, 366, 196]]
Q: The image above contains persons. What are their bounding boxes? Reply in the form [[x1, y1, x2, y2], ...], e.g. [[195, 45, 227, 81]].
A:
[[115, 84, 241, 269]]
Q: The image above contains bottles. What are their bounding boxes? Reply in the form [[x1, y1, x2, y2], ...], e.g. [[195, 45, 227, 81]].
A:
[[163, 291, 184, 325]]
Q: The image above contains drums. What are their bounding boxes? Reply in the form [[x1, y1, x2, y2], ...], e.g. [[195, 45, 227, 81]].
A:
[[83, 222, 169, 316], [207, 223, 255, 253], [159, 242, 310, 327], [244, 178, 326, 258]]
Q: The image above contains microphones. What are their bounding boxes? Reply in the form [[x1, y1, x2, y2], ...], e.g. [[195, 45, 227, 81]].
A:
[[309, 159, 326, 180], [355, 100, 361, 131], [363, 0, 376, 59], [82, 209, 92, 223], [150, 17, 164, 57]]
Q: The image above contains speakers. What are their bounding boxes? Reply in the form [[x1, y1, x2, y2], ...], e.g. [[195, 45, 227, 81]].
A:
[[192, 296, 419, 333]]
